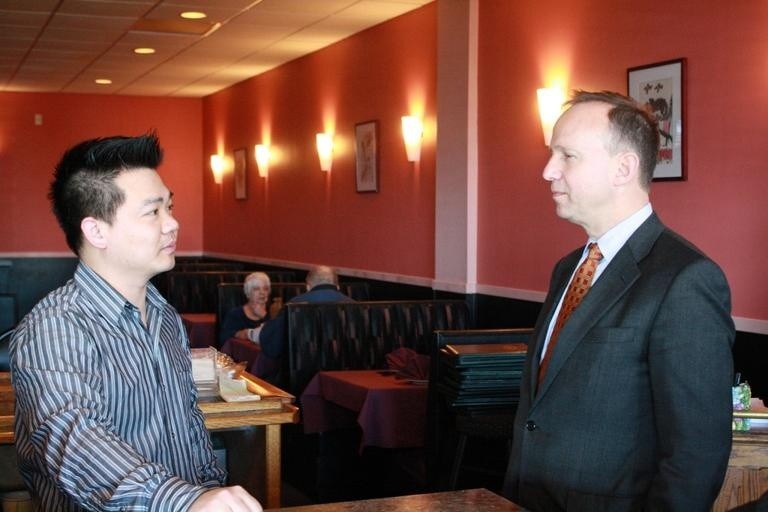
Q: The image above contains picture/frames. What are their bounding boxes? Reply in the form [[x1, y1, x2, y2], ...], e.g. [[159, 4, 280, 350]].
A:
[[354, 120, 379, 194], [233, 148, 247, 199], [627, 57, 686, 182]]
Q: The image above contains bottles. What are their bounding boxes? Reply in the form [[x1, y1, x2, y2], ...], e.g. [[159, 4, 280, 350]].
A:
[[732, 381, 752, 430]]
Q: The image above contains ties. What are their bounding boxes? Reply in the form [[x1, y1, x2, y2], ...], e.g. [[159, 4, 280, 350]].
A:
[[536, 242, 604, 381]]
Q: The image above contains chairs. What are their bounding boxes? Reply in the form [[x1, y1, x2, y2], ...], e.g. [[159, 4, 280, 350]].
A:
[[169, 271, 296, 313]]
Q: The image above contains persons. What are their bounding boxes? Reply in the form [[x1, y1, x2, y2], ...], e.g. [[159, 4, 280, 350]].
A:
[[504, 88, 736, 511], [9, 128, 266, 512], [259, 265, 359, 400], [218, 272, 274, 349]]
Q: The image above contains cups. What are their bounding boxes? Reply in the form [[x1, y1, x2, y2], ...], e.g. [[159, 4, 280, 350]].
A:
[[248, 329, 260, 343]]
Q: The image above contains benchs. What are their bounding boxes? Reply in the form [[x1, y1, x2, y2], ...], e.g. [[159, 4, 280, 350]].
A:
[[426, 327, 542, 481], [287, 301, 453, 377], [219, 283, 349, 333], [157, 262, 244, 297]]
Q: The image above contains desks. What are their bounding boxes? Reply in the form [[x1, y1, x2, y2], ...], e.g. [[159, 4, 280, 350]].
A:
[[0, 346, 299, 508], [220, 337, 262, 369], [713, 431, 768, 512], [264, 488, 529, 511], [298, 369, 432, 478], [178, 313, 216, 339]]
